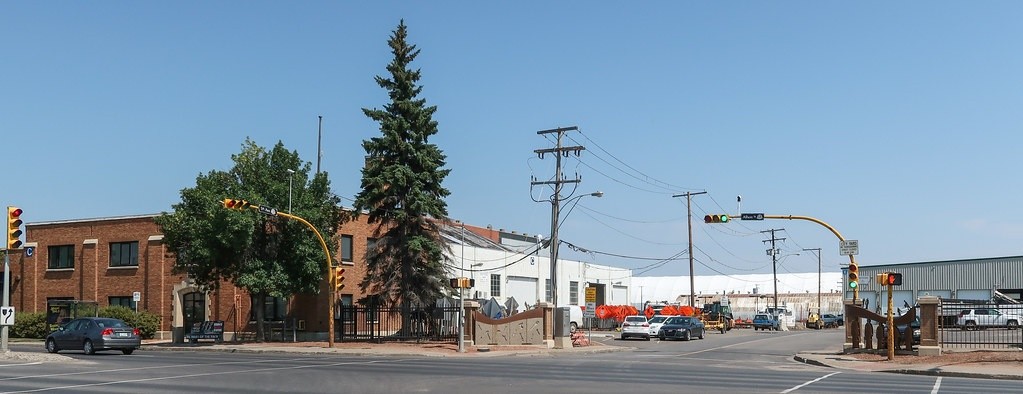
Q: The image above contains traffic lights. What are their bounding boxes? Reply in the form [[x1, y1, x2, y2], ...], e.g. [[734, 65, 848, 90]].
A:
[[450, 279, 461, 288], [704, 214, 729, 223], [6, 206, 23, 251], [466, 279, 475, 287], [888, 273, 902, 286], [848, 263, 858, 289], [335, 266, 345, 292], [224, 199, 250, 211]]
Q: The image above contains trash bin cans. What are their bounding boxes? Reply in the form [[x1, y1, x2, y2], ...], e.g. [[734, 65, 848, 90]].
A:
[[172, 327, 184, 343]]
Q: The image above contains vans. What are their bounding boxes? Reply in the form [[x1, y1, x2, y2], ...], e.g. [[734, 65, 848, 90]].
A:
[[529, 304, 584, 333]]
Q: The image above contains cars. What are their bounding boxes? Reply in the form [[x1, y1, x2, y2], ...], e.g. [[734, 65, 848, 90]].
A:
[[883, 315, 920, 348], [658, 316, 705, 341], [620, 315, 651, 341], [648, 315, 673, 338], [45, 317, 142, 356], [806, 312, 844, 330]]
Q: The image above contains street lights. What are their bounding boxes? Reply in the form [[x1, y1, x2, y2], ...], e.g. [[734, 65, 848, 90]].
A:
[[455, 219, 468, 353], [773, 253, 801, 312], [551, 191, 604, 340]]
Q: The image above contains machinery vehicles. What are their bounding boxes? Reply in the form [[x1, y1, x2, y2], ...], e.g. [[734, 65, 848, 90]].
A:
[[700, 300, 735, 334]]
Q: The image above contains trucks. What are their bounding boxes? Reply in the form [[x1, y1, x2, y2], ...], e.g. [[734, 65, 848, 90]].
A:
[[765, 305, 795, 331], [644, 302, 666, 316]]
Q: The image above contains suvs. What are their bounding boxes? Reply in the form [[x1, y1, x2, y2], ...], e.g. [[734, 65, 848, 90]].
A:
[[956, 309, 1023, 331], [752, 313, 780, 332]]
